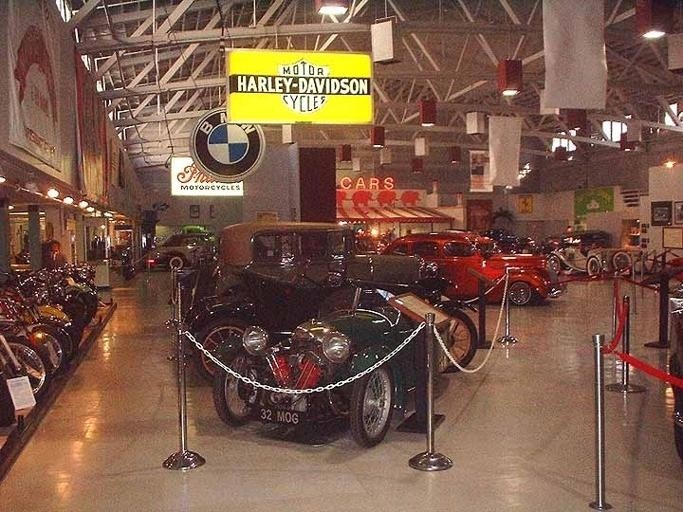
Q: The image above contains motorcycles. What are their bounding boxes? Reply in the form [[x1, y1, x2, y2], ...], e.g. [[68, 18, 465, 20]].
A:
[[0, 258, 100, 425]]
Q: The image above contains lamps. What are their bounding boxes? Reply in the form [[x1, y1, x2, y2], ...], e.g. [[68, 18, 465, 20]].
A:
[[372, 123, 388, 148], [621, 132, 636, 153], [415, 134, 429, 158], [464, 81, 485, 135], [450, 146, 460, 163], [380, 147, 392, 166], [625, 118, 643, 143], [420, 96, 436, 127], [564, 108, 588, 130], [352, 158, 361, 173], [635, 2, 670, 39], [499, 34, 522, 95], [340, 143, 352, 162], [370, 2, 404, 65]]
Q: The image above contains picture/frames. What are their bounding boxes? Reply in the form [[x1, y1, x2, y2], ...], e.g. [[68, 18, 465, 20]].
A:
[[662, 201, 683, 249]]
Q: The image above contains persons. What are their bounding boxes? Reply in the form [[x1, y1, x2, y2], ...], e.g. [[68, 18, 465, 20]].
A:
[[45, 239, 66, 268]]
